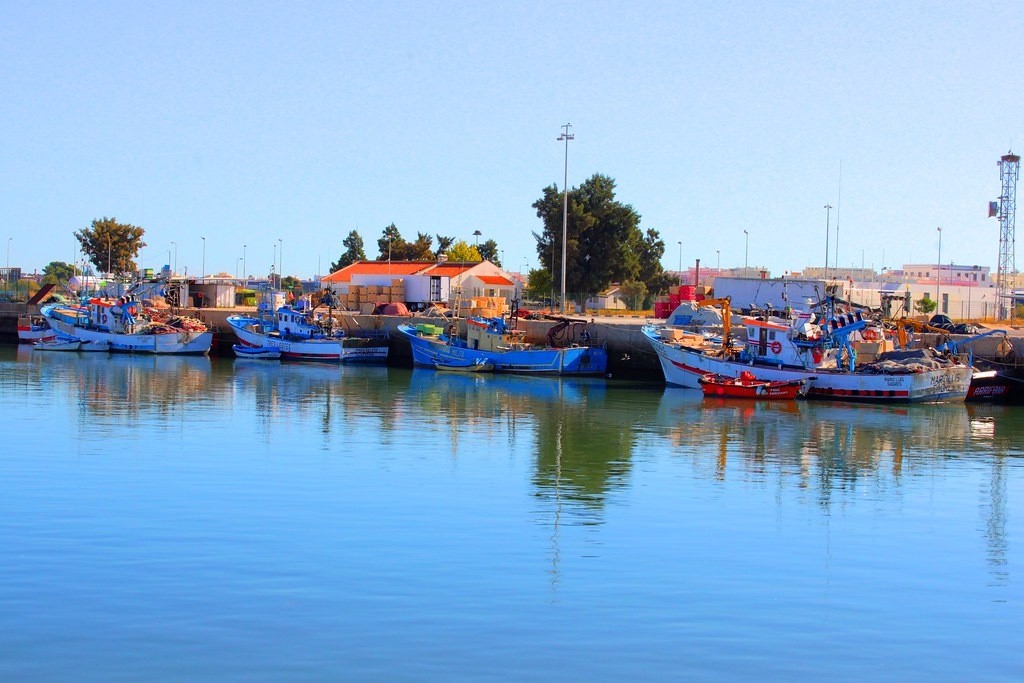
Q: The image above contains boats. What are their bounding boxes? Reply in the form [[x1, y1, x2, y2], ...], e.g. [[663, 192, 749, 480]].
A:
[[641, 297, 973, 404], [697, 370, 809, 400], [435, 362, 495, 373], [225, 283, 390, 362], [17, 297, 214, 356], [396, 256, 608, 377], [232, 343, 281, 360]]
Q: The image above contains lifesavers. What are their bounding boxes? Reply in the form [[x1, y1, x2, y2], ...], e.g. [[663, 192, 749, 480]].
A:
[[771, 341, 781, 354], [102, 314, 107, 321], [865, 329, 876, 339]]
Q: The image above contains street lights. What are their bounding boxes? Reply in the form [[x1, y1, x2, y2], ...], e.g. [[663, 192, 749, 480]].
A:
[[200, 237, 205, 278], [823, 205, 832, 282], [716, 250, 720, 273], [272, 245, 276, 289], [677, 241, 683, 288], [6, 237, 12, 304], [278, 239, 283, 292], [742, 229, 749, 268], [936, 226, 943, 318], [556, 121, 575, 315], [387, 234, 393, 286], [242, 245, 247, 286]]
